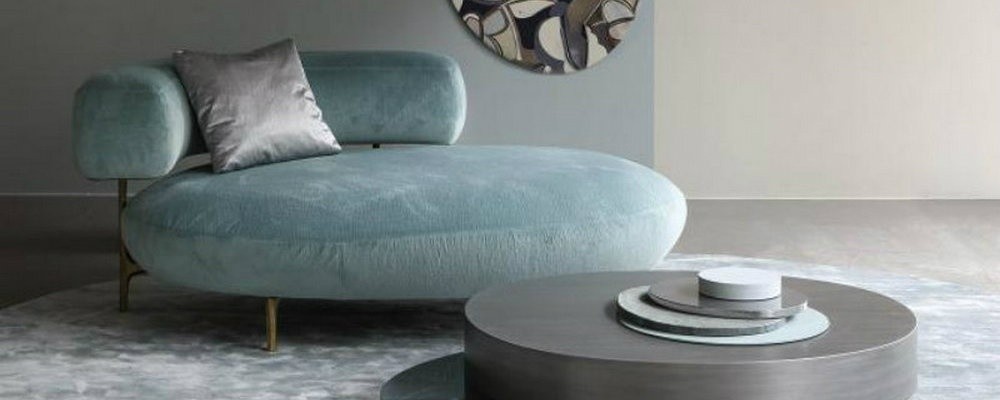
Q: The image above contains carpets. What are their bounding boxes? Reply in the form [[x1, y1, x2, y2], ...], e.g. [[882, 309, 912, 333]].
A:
[[1, 253, 1000, 400]]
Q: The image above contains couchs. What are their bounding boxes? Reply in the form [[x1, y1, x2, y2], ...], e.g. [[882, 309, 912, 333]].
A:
[[72, 50, 690, 352]]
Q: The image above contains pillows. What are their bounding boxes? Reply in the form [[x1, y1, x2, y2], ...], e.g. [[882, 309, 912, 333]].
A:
[[169, 36, 343, 174]]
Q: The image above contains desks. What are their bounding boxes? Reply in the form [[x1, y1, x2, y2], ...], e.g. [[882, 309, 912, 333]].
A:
[[463, 272, 917, 400]]
